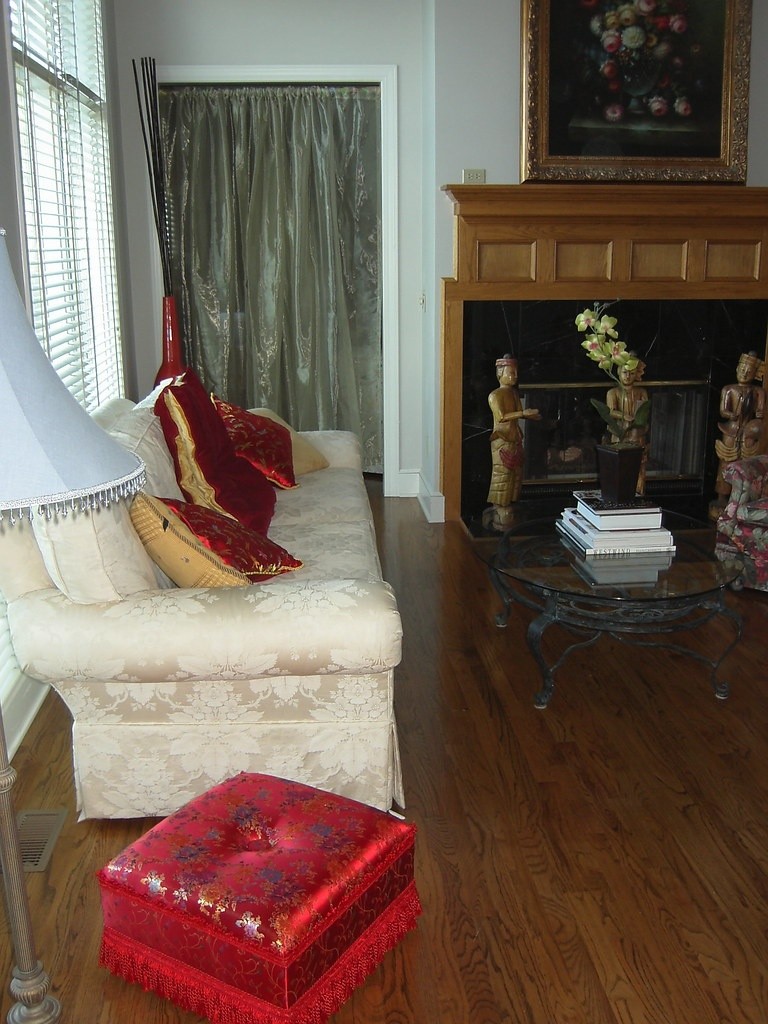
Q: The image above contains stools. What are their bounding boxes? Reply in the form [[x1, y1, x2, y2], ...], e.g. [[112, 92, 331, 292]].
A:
[[96, 768, 426, 1024]]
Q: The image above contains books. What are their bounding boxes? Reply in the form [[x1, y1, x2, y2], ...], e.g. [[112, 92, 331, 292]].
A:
[[555, 490, 676, 555], [570, 553, 672, 589]]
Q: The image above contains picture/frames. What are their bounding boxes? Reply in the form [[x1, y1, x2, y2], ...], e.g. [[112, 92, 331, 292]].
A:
[[519, 0, 753, 185]]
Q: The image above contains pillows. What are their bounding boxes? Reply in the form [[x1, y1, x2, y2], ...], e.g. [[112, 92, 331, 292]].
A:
[[130, 376, 174, 409], [154, 367, 278, 539], [30, 407, 184, 604], [244, 407, 330, 475], [153, 495, 304, 583], [209, 393, 295, 489], [129, 491, 253, 588]]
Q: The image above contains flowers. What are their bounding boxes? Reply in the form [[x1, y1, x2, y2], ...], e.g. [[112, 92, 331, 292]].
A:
[[575, 295, 658, 442]]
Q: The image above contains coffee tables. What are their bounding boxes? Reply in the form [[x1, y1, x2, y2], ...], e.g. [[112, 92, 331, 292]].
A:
[[469, 508, 746, 709]]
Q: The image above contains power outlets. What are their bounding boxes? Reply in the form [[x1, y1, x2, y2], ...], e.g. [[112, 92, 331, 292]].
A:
[[462, 168, 485, 183]]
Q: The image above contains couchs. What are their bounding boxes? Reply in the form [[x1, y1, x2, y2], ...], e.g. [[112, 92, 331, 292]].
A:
[[0, 397, 404, 822], [717, 456, 768, 592]]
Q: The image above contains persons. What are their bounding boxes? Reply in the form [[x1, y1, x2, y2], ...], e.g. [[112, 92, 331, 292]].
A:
[[606, 350, 648, 494], [487, 354, 541, 507], [715, 350, 765, 496]]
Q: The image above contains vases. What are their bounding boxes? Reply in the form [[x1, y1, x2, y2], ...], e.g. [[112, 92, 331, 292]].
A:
[[596, 442, 646, 501]]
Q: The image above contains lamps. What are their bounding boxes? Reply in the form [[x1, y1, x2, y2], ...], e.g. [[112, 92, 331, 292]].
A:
[[0, 226, 147, 1024]]
